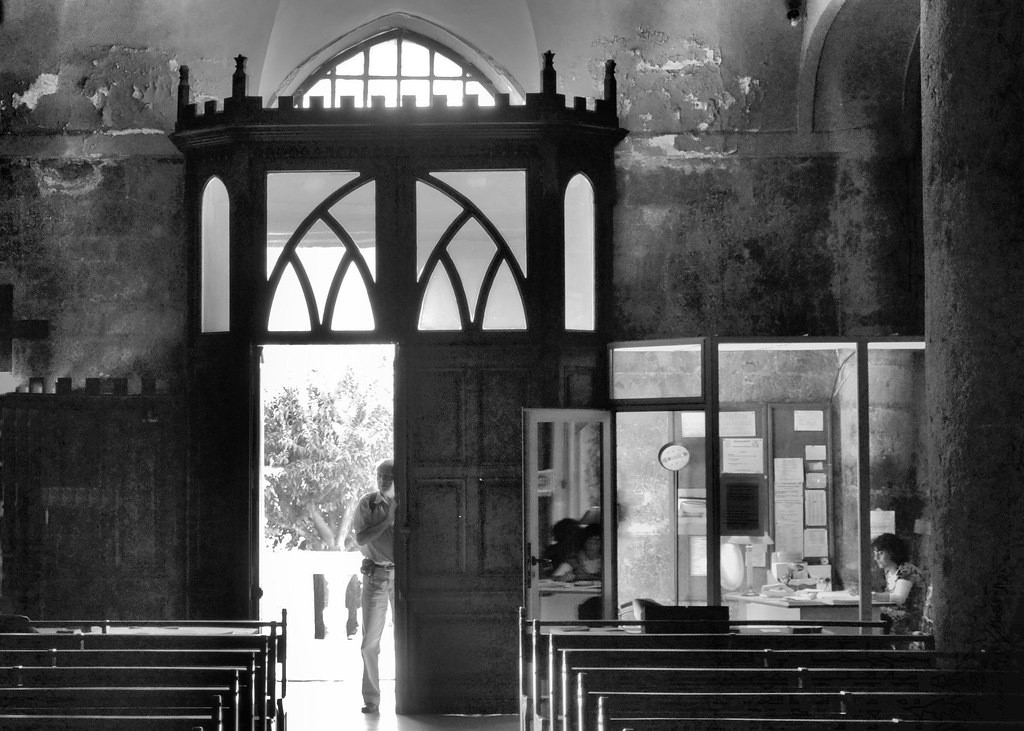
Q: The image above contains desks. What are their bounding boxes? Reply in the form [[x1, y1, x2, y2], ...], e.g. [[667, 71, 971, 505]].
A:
[[724, 594, 897, 635]]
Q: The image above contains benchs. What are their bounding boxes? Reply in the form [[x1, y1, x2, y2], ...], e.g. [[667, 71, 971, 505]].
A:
[[0, 608, 288, 731], [518, 606, 1024, 731]]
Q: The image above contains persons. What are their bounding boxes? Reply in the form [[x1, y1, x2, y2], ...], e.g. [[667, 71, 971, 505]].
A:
[[847, 532, 927, 633], [549, 529, 604, 582], [353, 459, 398, 712]]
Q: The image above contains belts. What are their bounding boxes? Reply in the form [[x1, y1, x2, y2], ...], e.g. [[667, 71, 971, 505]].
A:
[[375, 563, 394, 571]]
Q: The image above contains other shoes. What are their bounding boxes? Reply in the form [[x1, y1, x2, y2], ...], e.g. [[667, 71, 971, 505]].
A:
[[362, 703, 378, 713]]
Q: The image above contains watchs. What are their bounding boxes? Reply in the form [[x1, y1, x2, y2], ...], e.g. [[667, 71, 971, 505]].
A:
[[871, 591, 876, 599]]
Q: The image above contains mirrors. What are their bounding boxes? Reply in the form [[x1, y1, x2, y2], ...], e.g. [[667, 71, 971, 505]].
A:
[[535, 419, 604, 594]]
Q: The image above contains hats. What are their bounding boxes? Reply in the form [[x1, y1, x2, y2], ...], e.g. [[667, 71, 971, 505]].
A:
[[376, 459, 393, 477]]
